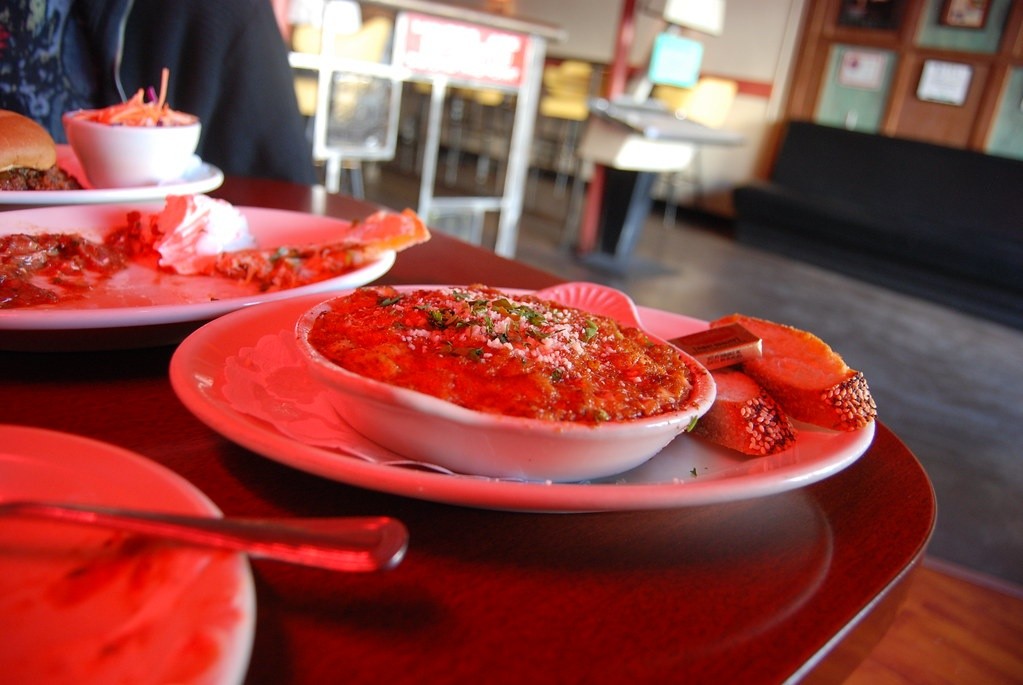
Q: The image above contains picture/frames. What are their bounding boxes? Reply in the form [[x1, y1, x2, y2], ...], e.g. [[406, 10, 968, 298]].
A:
[[938, 0, 993, 30]]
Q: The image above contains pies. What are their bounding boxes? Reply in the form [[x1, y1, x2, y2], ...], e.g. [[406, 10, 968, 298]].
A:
[[328, 206, 430, 271]]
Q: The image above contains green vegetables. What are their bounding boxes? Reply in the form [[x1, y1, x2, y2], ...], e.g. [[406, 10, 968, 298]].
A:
[[378, 289, 600, 382]]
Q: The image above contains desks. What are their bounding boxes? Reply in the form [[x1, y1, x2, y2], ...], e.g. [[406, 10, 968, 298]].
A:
[[545, 53, 772, 97]]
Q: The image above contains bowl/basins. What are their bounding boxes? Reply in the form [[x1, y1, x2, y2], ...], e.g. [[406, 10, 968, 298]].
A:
[[296, 284, 717, 484], [0, 422, 257, 685], [62, 108, 202, 188]]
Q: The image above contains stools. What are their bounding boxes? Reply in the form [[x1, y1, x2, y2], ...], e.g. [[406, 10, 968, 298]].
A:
[[289, 17, 738, 235]]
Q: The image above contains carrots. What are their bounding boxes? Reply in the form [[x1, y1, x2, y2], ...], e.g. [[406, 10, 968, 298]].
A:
[[70, 67, 179, 127]]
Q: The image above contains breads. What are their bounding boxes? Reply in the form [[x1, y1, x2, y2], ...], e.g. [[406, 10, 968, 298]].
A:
[[683, 312, 877, 457], [0, 109, 57, 173]]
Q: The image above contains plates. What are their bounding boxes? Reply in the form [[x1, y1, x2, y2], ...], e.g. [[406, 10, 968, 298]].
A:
[[0, 203, 398, 353], [170, 284, 876, 513], [0, 144, 224, 206]]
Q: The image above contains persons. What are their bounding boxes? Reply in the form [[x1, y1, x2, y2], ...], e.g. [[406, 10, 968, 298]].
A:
[[0, 0, 317, 184]]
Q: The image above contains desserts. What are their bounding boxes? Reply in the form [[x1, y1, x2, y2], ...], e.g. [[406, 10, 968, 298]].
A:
[[152, 189, 257, 274]]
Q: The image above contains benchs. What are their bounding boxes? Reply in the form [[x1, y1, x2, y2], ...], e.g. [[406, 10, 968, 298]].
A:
[[729, 119, 1023, 335]]
